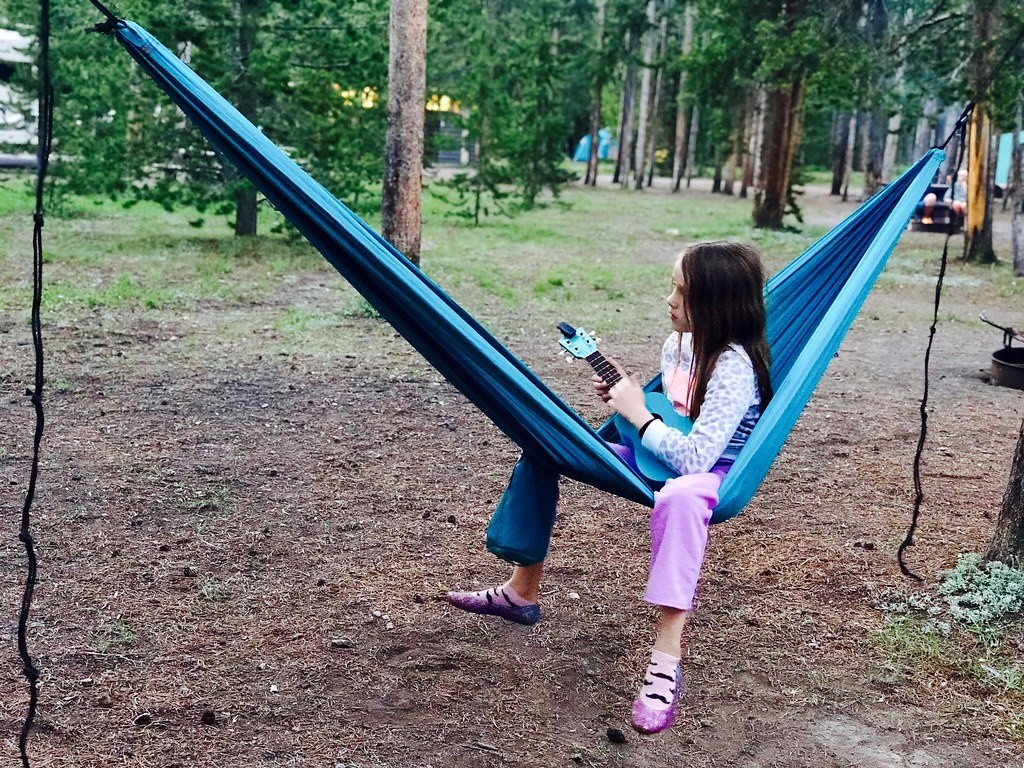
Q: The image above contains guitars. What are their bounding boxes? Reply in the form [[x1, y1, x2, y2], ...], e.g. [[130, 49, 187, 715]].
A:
[[553, 318, 697, 484]]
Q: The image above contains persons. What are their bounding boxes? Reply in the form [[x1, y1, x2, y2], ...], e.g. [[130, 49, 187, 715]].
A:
[[914, 171, 968, 234], [447, 241, 773, 734]]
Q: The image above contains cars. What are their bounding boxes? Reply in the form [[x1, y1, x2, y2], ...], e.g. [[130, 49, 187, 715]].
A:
[[907, 184, 965, 232], [946, 170, 1003, 200]]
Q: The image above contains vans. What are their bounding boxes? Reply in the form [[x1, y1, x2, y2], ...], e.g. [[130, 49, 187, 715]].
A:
[[1, 29, 73, 169]]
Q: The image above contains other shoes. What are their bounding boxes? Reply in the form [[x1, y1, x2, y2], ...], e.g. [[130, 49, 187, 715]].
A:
[[630, 662, 681, 734], [447, 586, 540, 626]]
